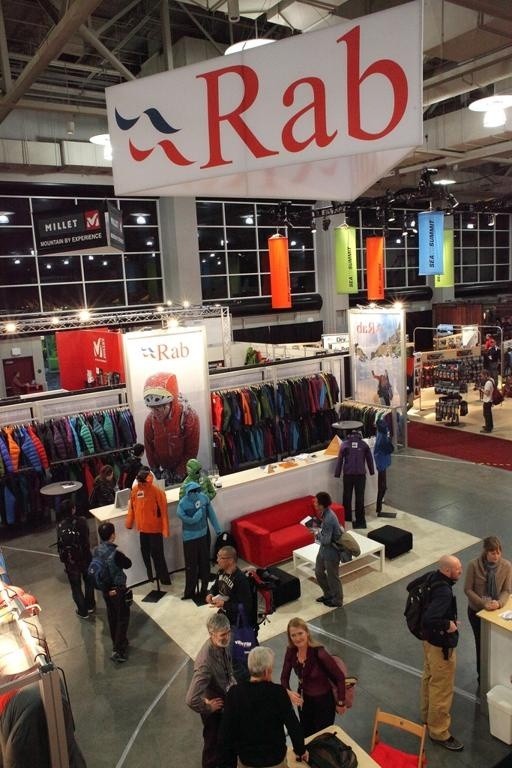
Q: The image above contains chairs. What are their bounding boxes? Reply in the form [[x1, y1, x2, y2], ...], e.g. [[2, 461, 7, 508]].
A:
[[369, 704, 428, 768]]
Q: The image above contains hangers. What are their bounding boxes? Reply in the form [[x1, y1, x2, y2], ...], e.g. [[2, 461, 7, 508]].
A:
[[210, 370, 331, 404], [1, 400, 131, 437]]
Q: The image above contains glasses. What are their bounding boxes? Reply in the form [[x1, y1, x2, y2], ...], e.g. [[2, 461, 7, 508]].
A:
[[148, 405, 168, 412], [217, 553, 232, 560]]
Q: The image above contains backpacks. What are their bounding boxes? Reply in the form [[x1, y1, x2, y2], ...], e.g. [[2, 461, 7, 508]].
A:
[[327, 655, 359, 709], [404, 571, 453, 641], [56, 516, 86, 564], [331, 528, 361, 557], [87, 546, 114, 592]]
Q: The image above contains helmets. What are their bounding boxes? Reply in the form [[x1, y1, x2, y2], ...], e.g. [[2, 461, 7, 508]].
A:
[[143, 392, 172, 407]]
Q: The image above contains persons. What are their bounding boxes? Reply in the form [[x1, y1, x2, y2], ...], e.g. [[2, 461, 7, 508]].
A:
[[463, 536, 512, 685], [125, 459, 222, 603], [55, 499, 133, 664], [421, 554, 464, 751], [474, 370, 496, 434], [10, 370, 25, 396], [143, 371, 200, 487], [310, 492, 344, 607], [334, 422, 394, 528], [371, 369, 391, 406], [186, 545, 346, 768], [94, 443, 144, 508], [485, 333, 512, 377]]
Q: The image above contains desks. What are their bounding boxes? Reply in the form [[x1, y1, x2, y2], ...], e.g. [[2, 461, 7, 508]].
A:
[[284, 723, 384, 768]]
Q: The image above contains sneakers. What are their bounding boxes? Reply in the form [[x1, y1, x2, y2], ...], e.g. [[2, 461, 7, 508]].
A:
[[427, 735, 464, 750], [75, 608, 89, 619], [88, 605, 96, 613], [109, 650, 128, 665]]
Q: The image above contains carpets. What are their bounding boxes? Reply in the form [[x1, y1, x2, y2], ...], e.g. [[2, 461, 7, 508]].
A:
[[129, 499, 484, 660]]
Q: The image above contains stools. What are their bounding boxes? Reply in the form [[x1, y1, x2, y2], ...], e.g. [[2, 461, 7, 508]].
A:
[[260, 567, 300, 606], [368, 525, 412, 560]]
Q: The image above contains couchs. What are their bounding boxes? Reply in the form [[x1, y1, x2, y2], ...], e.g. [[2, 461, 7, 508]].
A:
[[230, 495, 344, 571]]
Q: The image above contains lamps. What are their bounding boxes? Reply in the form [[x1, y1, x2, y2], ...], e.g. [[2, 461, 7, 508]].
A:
[[468, 93, 512, 128], [90, 133, 113, 160], [283, 179, 495, 245]]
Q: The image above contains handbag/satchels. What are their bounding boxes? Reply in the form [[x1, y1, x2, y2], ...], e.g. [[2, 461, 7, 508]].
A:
[[231, 603, 258, 666], [486, 379, 504, 405], [303, 731, 358, 768]]
